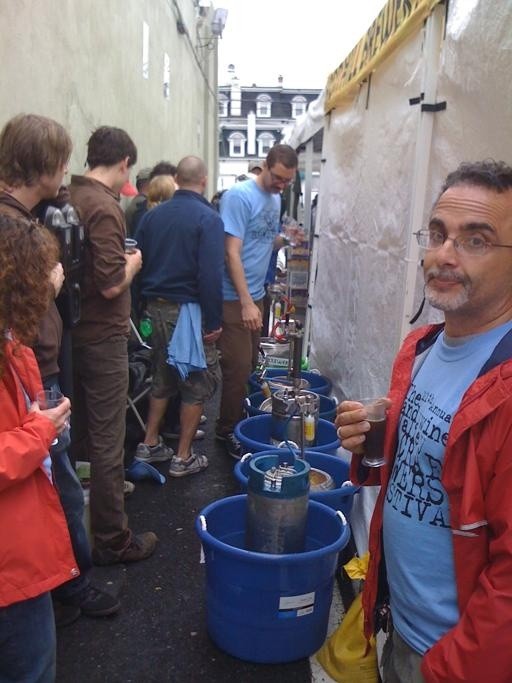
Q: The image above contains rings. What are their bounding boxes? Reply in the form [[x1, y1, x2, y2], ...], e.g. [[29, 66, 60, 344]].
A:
[[336, 426, 344, 440]]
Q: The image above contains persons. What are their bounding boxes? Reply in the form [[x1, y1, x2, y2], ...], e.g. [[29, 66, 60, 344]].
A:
[[334, 155, 509, 682], [67, 124, 162, 567], [125, 142, 305, 479], [1, 204, 80, 683], [0, 113, 121, 631]]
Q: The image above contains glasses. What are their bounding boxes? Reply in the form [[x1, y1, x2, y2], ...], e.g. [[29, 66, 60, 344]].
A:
[[414, 228, 512, 255]]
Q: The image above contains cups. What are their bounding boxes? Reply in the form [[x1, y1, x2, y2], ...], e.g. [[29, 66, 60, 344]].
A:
[[356, 396, 387, 467], [287, 222, 304, 247], [36, 389, 63, 412], [124, 237, 138, 253]]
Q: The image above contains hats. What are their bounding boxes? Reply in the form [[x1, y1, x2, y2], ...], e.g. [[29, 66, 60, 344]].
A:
[[248, 159, 264, 173]]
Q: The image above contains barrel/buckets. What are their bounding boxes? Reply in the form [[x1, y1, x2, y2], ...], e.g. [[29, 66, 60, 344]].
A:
[[189, 492, 352, 667], [256, 356, 309, 371], [243, 390, 338, 423], [234, 412, 342, 458], [247, 368, 334, 397], [230, 448, 361, 524]]
